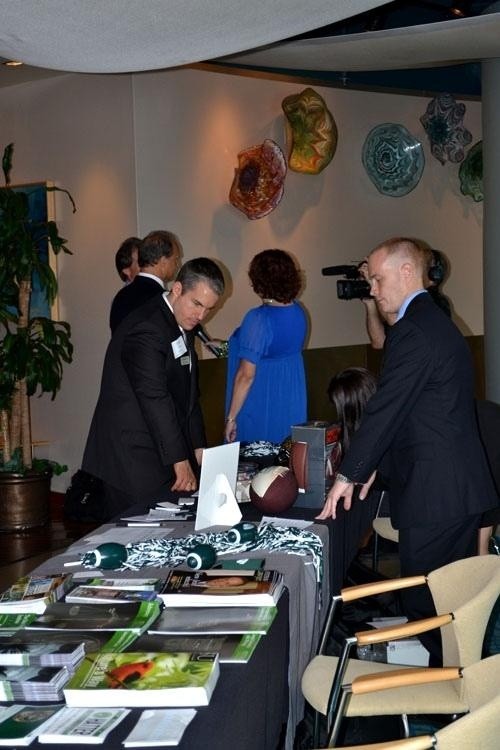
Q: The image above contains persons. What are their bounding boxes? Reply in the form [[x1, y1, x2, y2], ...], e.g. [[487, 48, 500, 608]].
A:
[[204, 246, 309, 448], [114, 236, 143, 284], [316, 237, 499, 618], [107, 228, 184, 337], [327, 364, 380, 462], [355, 239, 452, 381], [77, 255, 226, 524]]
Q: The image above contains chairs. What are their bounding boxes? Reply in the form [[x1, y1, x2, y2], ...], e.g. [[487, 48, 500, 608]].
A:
[[372, 489, 400, 581], [302, 553, 500, 738], [327, 654, 500, 750]]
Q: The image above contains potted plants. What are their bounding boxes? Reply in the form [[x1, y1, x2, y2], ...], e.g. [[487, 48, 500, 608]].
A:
[[1, 141, 76, 531]]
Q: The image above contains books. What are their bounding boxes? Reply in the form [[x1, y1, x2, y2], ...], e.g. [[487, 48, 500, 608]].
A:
[[0, 558, 287, 750]]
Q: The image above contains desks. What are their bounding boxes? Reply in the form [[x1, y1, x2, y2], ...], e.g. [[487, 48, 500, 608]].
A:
[[0, 474, 387, 750]]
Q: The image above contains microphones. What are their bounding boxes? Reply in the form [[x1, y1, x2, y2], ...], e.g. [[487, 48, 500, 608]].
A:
[[191, 322, 223, 358]]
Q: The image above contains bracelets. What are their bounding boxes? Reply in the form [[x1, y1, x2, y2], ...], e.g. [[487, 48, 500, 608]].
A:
[[335, 473, 352, 483], [219, 340, 228, 358], [226, 417, 236, 422]]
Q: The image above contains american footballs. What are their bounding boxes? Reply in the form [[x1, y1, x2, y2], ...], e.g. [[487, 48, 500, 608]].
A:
[[250, 465, 298, 513]]
